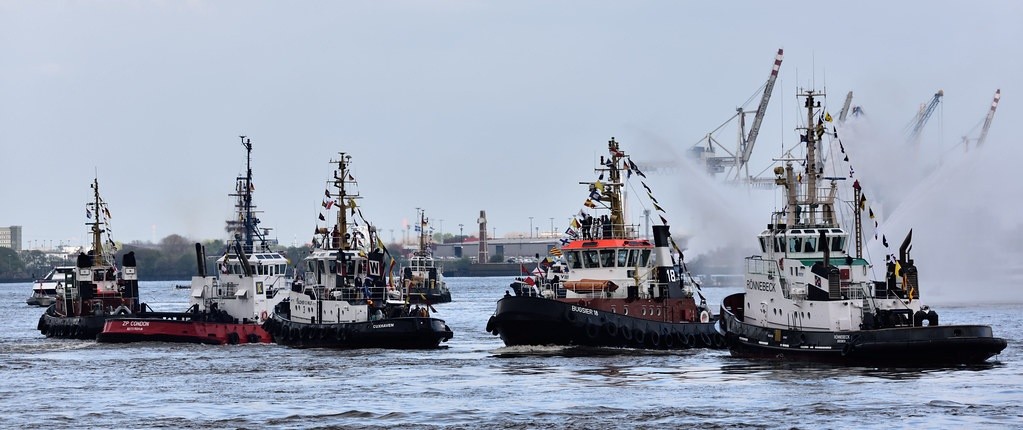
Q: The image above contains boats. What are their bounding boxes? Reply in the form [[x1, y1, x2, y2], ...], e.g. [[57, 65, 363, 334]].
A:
[[42, 177, 146, 340], [496, 135, 723, 348], [271, 152, 445, 349], [96, 134, 292, 344], [713, 48, 1008, 367], [26, 255, 77, 306], [388, 207, 451, 303]]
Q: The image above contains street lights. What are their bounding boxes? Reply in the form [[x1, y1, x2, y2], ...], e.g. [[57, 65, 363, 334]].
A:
[[377, 215, 576, 244]]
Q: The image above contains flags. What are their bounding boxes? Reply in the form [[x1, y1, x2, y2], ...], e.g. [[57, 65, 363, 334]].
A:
[[795, 112, 916, 302], [86, 206, 118, 251], [311, 174, 415, 309], [522, 148, 706, 302]]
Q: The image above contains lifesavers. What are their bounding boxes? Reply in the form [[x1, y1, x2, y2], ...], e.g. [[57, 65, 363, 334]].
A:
[[260, 318, 349, 343], [248, 333, 259, 343], [261, 310, 267, 320], [486, 315, 725, 349], [45, 324, 88, 339], [229, 332, 240, 345]]
